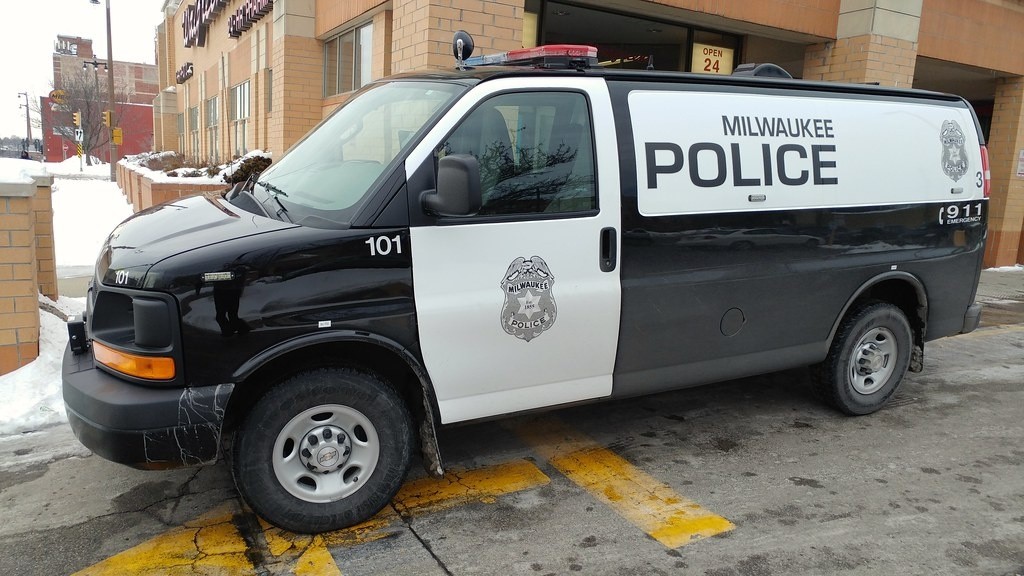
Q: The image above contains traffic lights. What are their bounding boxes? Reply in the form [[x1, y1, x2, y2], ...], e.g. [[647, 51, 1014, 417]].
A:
[[113, 129, 122, 146], [73, 112, 81, 128], [101, 111, 110, 128]]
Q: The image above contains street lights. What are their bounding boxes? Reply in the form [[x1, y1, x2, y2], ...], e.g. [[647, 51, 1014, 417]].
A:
[[80, 60, 108, 164], [90, 0, 117, 182], [18, 92, 30, 140], [19, 105, 32, 146]]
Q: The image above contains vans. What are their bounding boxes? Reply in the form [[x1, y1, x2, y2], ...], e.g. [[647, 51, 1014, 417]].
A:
[[59, 30, 990, 533]]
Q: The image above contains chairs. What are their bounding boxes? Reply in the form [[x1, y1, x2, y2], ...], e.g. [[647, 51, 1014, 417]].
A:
[[444, 106, 584, 215]]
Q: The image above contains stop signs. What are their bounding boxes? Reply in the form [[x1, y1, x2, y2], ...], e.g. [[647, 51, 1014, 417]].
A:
[[62, 145, 67, 151]]
